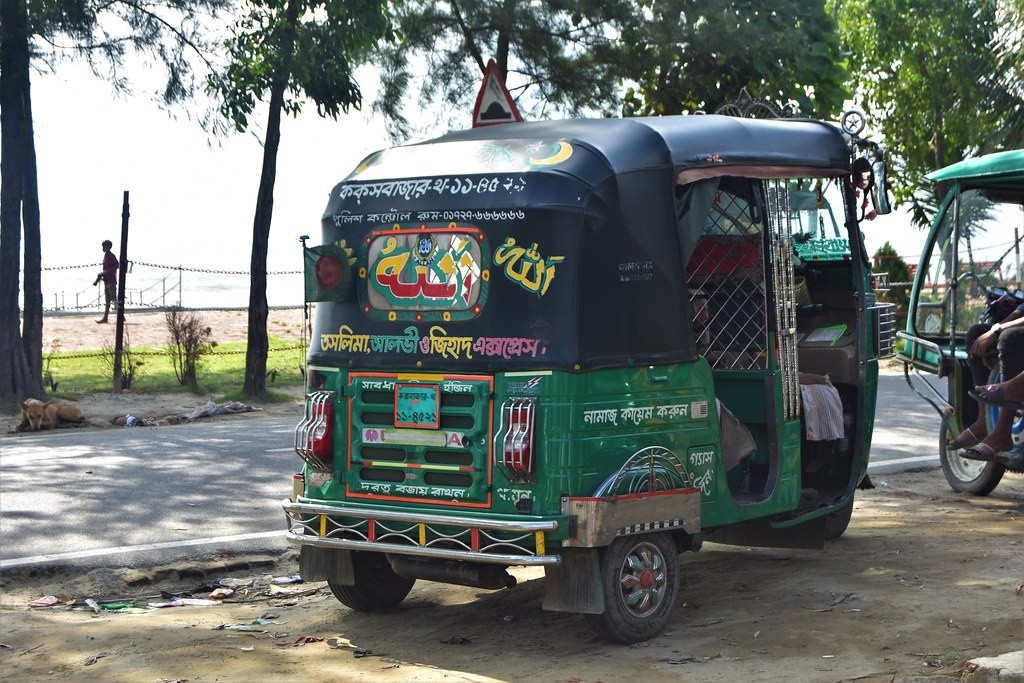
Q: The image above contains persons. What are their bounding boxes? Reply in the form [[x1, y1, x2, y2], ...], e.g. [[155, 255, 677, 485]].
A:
[[945, 302, 1024, 462], [94, 239, 126, 325]]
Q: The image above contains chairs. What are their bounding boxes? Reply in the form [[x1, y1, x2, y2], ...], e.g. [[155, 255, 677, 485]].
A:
[[694, 272, 776, 354]]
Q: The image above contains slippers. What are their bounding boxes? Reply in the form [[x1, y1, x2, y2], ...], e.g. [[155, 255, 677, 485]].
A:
[[958, 443, 1008, 464], [944, 428, 986, 450], [965, 384, 1023, 411]]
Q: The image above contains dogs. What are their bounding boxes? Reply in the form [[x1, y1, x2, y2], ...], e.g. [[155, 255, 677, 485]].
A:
[[8, 398, 92, 433]]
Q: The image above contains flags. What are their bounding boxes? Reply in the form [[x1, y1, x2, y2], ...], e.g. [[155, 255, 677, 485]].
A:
[[302, 243, 362, 305]]
